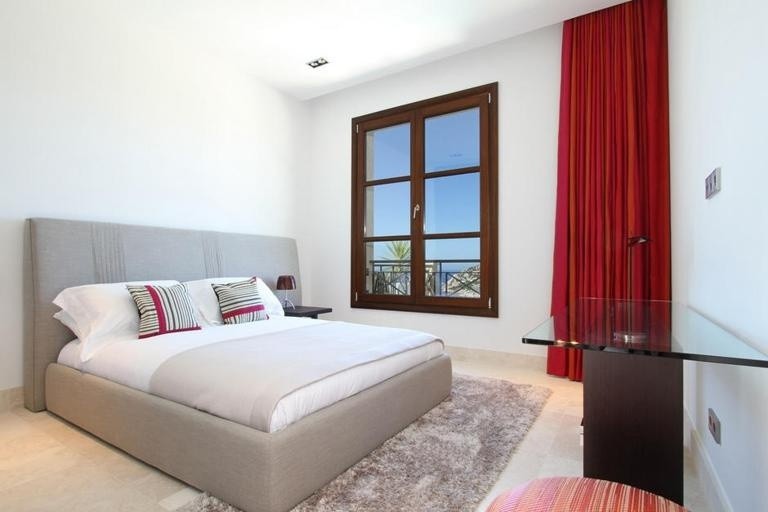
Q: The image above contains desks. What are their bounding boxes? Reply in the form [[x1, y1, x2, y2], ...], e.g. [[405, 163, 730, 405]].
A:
[[519, 296, 768, 506]]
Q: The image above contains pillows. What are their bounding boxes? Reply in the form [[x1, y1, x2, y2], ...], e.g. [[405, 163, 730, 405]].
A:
[[51, 276, 286, 363]]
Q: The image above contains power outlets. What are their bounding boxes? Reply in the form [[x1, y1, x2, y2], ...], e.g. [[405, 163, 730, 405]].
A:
[[706, 407, 720, 444]]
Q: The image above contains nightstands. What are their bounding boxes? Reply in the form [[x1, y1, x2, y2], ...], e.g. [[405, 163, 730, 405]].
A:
[[283, 306, 333, 319]]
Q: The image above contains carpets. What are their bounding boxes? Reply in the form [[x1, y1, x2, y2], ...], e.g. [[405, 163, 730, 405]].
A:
[[175, 372, 555, 511]]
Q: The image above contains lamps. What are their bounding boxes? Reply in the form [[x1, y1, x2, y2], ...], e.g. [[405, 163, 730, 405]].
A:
[[276, 275, 297, 311]]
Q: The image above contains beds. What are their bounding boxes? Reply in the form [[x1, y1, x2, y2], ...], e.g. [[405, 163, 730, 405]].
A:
[[24, 218, 453, 511]]
